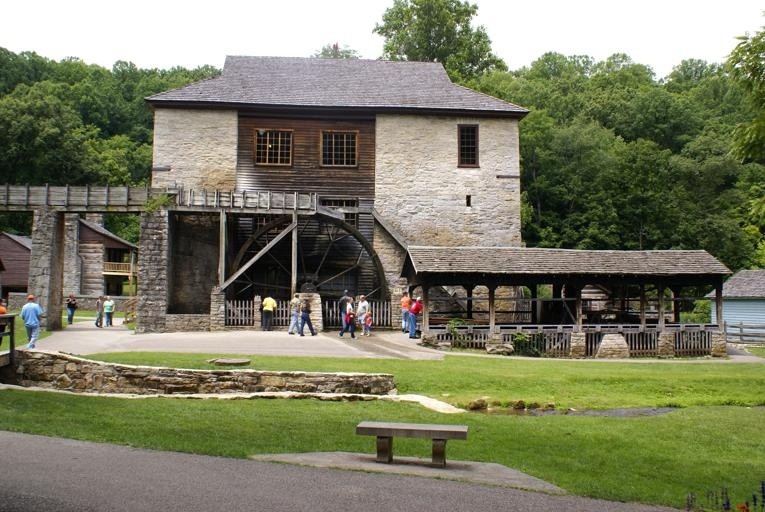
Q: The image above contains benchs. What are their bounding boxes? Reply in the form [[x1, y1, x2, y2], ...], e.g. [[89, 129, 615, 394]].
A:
[[355, 420, 469, 468]]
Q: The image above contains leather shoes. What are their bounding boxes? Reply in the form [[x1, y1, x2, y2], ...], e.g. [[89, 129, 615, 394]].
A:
[[289, 332, 317, 336], [402, 328, 420, 339]]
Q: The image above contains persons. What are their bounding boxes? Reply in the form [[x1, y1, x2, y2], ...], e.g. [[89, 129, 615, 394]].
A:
[[261, 296, 278, 330], [94, 295, 116, 328], [0, 293, 44, 350], [337, 290, 373, 339], [289, 293, 317, 336], [401, 291, 422, 338], [66, 292, 76, 324]]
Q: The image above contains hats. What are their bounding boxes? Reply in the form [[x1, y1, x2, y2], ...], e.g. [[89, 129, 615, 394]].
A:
[[28, 294, 34, 299]]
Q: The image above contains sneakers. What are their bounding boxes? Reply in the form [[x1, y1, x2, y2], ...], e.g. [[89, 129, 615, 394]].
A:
[[338, 332, 370, 338]]
